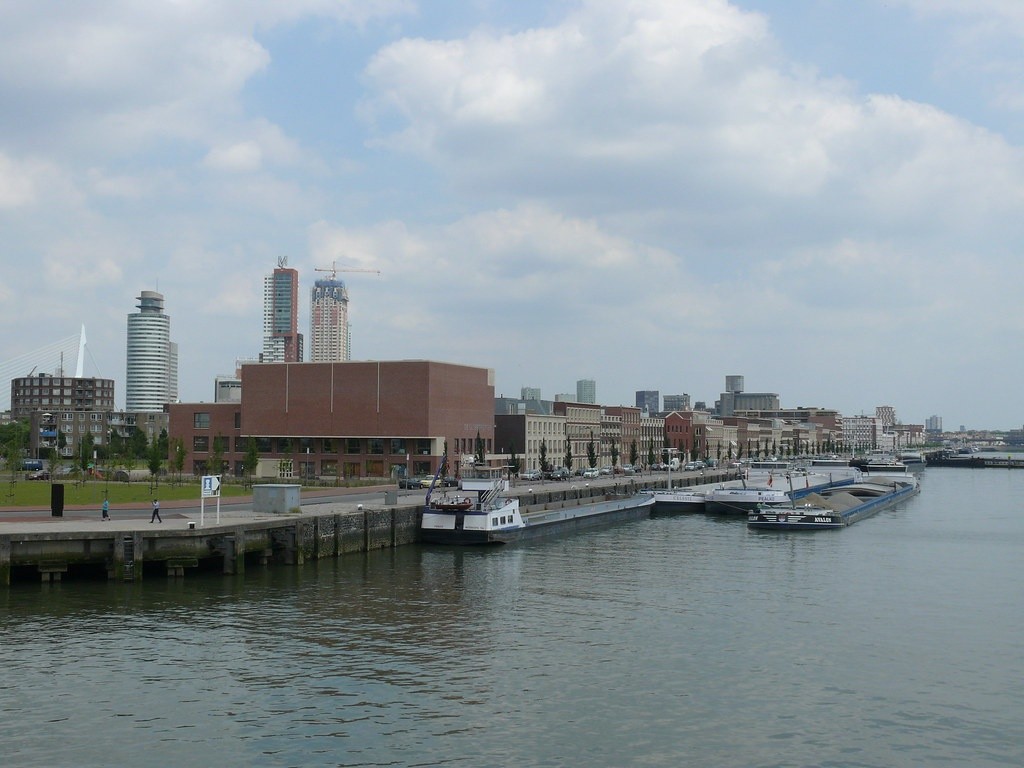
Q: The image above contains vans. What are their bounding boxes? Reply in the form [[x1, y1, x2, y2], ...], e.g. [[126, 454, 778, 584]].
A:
[[16, 459, 43, 472]]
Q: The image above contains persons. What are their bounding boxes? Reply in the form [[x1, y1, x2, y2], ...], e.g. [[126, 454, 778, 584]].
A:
[[149, 499, 162, 524], [240, 464, 244, 477], [101, 497, 111, 521], [767, 472, 773, 488], [456, 475, 461, 487], [786, 474, 790, 484]]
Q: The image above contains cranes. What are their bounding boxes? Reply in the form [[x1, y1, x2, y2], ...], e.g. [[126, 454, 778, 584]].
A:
[[314, 260, 381, 281]]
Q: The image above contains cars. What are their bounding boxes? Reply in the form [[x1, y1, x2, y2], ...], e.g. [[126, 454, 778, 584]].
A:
[[520, 468, 541, 481], [28, 469, 51, 481], [439, 476, 458, 487], [399, 476, 423, 491], [420, 474, 441, 489], [53, 464, 83, 478], [539, 455, 779, 483]]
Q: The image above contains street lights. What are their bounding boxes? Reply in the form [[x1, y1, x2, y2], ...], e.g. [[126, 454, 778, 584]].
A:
[[93, 449, 97, 504], [406, 453, 410, 498], [176, 446, 179, 485], [305, 447, 310, 488]]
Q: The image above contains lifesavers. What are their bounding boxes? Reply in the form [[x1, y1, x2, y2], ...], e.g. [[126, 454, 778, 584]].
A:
[[505, 498, 512, 505], [463, 498, 471, 504]]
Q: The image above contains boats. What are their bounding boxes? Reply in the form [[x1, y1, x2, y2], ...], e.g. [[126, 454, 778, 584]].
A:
[[850, 456, 909, 475], [748, 475, 921, 532], [420, 453, 656, 545], [705, 470, 856, 514], [643, 450, 779, 513]]
